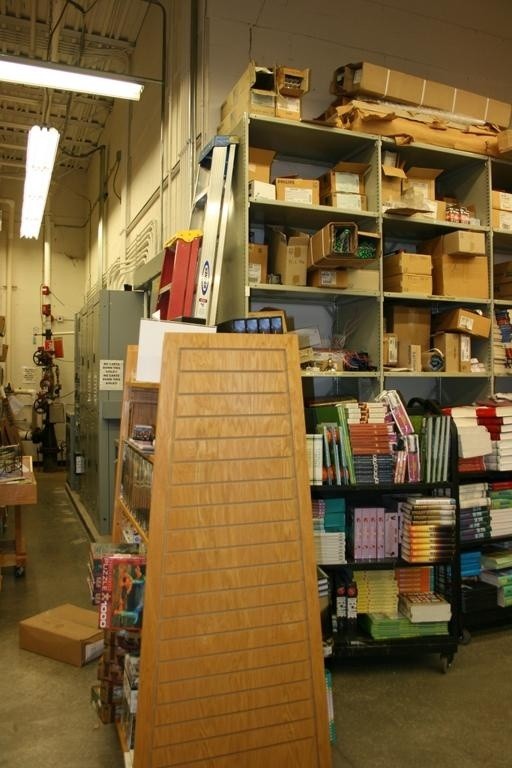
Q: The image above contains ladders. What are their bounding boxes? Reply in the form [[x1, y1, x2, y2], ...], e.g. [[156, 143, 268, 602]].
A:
[[156, 232, 201, 321], [188, 134, 242, 326]]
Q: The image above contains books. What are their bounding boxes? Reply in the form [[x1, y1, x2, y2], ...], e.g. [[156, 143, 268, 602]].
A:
[[493, 307, 512, 369], [457, 479, 512, 541], [120, 447, 162, 533], [334, 568, 454, 636], [448, 396, 511, 474], [460, 541, 512, 611], [307, 390, 452, 485], [313, 494, 459, 567], [82, 539, 143, 633]]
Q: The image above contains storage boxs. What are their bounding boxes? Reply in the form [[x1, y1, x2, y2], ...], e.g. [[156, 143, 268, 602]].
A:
[[19, 602, 104, 667], [329, 60, 512, 151], [220, 63, 311, 120]]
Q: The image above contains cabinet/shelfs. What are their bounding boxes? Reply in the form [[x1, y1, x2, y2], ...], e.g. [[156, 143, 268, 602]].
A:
[[218, 113, 512, 404], [452, 415, 512, 635], [74, 291, 145, 541], [300, 397, 460, 669], [99, 334, 328, 767]]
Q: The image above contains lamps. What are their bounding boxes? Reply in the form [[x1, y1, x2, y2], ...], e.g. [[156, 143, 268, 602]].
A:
[[17, 121, 62, 239], [0, 54, 145, 104]]
